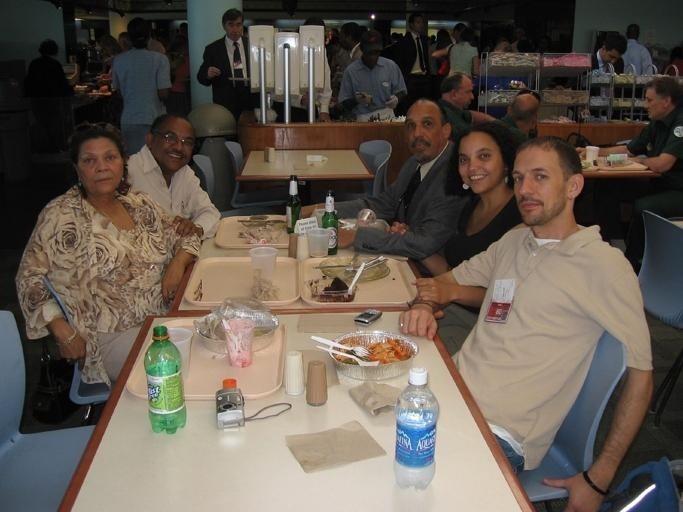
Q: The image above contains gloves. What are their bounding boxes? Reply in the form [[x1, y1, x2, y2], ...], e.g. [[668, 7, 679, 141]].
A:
[[385, 95, 398, 109], [355, 91, 372, 106]]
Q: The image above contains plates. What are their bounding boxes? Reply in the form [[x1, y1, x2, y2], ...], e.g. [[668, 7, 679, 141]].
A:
[[319, 256, 390, 283]]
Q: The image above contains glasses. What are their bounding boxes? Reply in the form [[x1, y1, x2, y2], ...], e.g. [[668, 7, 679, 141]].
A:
[[151, 131, 194, 149]]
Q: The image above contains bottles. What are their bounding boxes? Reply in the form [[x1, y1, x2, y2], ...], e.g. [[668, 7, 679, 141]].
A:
[[284, 351, 304, 396], [392, 366, 439, 490], [285, 175, 301, 234], [306, 361, 328, 406], [263, 147, 268, 162], [288, 233, 299, 258], [295, 235, 308, 259], [321, 190, 337, 255], [267, 148, 274, 163], [143, 326, 187, 433]]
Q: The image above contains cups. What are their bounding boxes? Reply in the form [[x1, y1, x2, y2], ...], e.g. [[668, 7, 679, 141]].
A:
[[356, 209, 375, 225], [247, 247, 279, 297], [221, 319, 256, 368], [150, 328, 193, 377], [306, 228, 329, 257], [585, 146, 599, 162], [306, 155, 327, 168]]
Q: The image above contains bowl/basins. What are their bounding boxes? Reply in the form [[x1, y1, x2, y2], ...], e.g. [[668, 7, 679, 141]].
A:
[[302, 278, 357, 302], [328, 329, 419, 381], [194, 310, 279, 354]]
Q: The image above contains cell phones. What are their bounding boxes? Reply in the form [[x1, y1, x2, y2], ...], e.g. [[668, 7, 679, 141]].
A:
[[354, 307, 382, 326]]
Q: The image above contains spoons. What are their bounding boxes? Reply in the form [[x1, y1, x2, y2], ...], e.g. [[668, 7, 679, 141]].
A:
[[313, 345, 379, 367]]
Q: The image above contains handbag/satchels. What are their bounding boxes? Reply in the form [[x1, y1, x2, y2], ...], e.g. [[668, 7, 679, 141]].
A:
[[33, 348, 82, 425], [437, 57, 449, 77]]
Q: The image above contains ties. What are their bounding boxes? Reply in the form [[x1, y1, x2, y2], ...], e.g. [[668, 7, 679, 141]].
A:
[[404, 165, 421, 217], [233, 41, 243, 88], [416, 37, 424, 72], [601, 65, 612, 98]]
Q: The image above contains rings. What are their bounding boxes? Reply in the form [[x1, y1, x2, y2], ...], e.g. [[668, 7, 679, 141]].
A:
[[167, 290, 177, 300], [398, 320, 404, 327]]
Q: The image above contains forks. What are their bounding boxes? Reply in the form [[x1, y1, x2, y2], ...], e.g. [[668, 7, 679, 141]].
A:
[[308, 335, 370, 358]]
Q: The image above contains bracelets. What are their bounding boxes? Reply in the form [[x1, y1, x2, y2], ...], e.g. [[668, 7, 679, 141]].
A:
[[581, 467, 613, 497], [51, 331, 82, 349], [406, 296, 440, 311]]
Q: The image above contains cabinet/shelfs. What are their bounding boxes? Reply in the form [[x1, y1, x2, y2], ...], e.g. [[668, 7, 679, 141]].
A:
[[477, 50, 680, 125]]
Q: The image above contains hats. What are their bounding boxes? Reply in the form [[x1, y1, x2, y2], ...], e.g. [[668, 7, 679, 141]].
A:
[[360, 30, 384, 52]]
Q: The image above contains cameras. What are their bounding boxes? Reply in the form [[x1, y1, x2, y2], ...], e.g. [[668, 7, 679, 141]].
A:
[[214, 386, 247, 430]]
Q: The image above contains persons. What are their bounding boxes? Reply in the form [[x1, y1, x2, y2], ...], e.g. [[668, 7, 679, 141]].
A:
[[124, 113, 223, 240], [663, 46, 683, 74], [300, 97, 479, 259], [198, 8, 654, 148], [388, 122, 523, 279], [398, 136, 655, 511], [576, 76, 682, 262], [15, 119, 202, 387], [21, 17, 188, 151]]
